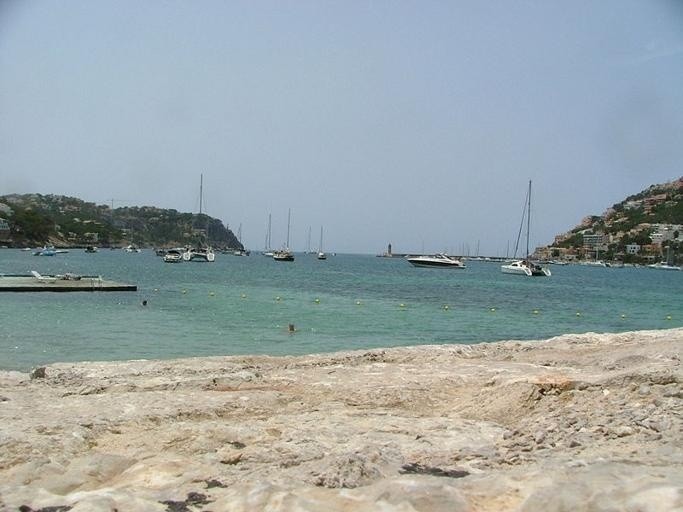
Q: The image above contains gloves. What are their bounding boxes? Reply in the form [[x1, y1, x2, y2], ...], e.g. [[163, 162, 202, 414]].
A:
[[458, 255, 519, 263], [406, 252, 467, 270], [537, 255, 682, 271]]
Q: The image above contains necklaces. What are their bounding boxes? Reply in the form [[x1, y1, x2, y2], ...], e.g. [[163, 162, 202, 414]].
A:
[[500, 179, 552, 277]]
